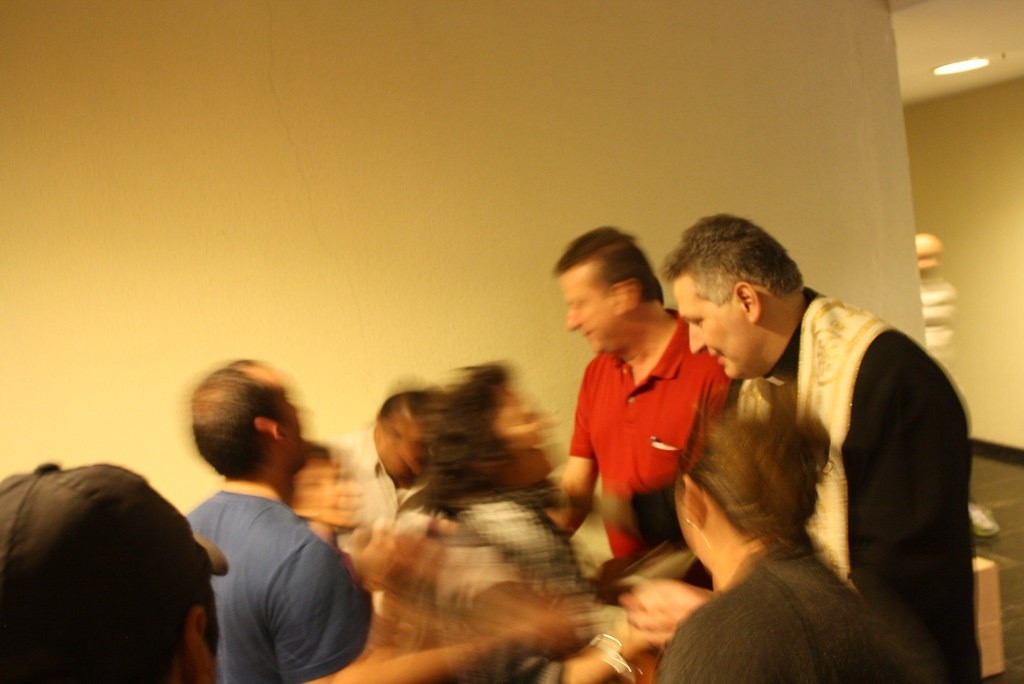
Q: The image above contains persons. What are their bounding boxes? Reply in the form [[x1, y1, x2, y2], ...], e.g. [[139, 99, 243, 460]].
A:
[[0, 214, 983, 684], [915, 232, 1000, 538]]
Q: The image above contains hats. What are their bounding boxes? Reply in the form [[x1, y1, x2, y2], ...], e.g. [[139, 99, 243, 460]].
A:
[[0, 461, 228, 684]]
